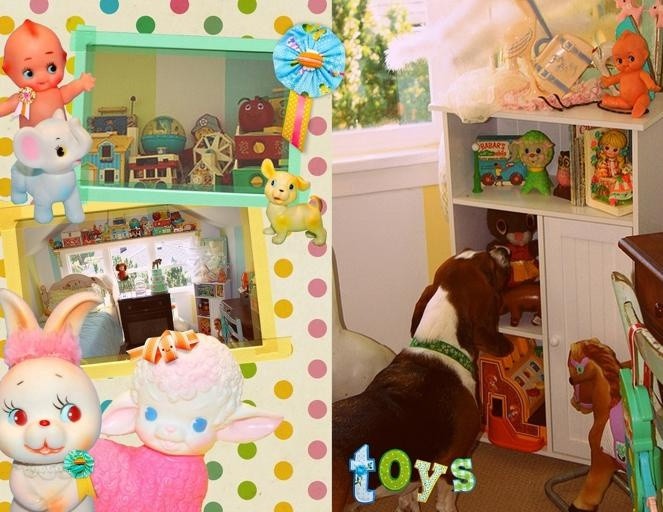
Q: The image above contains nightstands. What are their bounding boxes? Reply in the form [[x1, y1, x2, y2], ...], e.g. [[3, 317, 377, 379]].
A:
[[117, 290, 174, 349]]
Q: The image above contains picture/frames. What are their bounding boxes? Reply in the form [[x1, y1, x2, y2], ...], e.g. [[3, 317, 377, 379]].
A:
[[71, 23, 302, 207], [0, 201, 292, 381]]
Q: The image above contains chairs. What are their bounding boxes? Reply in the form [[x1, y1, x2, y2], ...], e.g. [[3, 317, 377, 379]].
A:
[[611, 271, 663, 451], [220, 310, 243, 344]]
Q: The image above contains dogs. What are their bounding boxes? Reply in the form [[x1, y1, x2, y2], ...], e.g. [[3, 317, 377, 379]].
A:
[[331, 245, 514, 511]]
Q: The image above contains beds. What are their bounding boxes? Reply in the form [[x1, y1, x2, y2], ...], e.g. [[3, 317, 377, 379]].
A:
[[39, 274, 123, 359]]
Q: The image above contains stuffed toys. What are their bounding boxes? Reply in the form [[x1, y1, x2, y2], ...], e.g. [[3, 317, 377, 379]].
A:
[[600, 29, 661, 119], [261, 158, 327, 246], [544, 339, 630, 512], [553, 149, 571, 202], [619, 368, 658, 511], [510, 129, 555, 197], [0, 19, 95, 224], [487, 207, 540, 290], [495, 282, 540, 326], [88, 329, 283, 511], [0, 288, 105, 511], [649, 0, 662, 30], [615, 0, 644, 29], [477, 334, 547, 455], [590, 130, 633, 207], [238, 96, 273, 134], [45, 211, 197, 249], [78, 94, 233, 185]]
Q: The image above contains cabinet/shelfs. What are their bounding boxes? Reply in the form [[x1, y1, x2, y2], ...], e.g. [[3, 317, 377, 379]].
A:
[[429, 92, 662, 474], [221, 292, 253, 342], [191, 279, 232, 343], [618, 231, 663, 344]]
